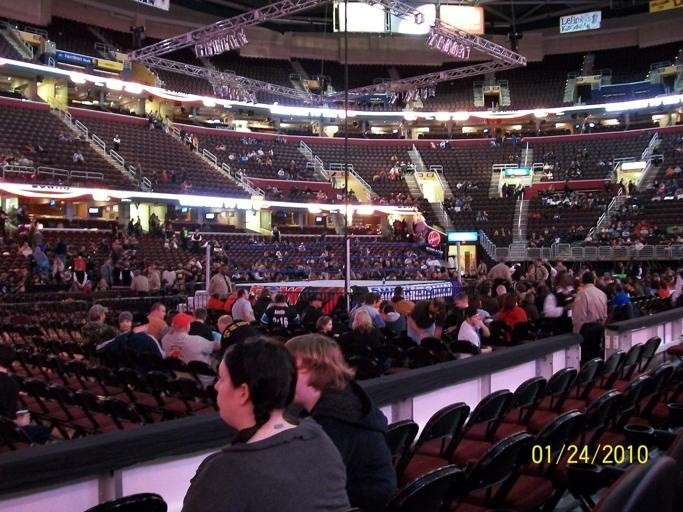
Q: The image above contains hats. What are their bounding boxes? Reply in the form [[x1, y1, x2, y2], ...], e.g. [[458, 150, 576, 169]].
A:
[[170, 312, 193, 328], [117, 310, 133, 323], [87, 304, 109, 319]]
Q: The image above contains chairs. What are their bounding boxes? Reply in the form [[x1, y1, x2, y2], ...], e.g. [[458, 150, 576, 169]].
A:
[[84, 492, 167, 512], [213, 236, 438, 281], [0, 232, 206, 285], [500, 78, 571, 110], [1, 102, 250, 197], [416, 88, 474, 112], [415, 133, 682, 246], [149, 67, 214, 96], [389, 337, 683, 511], [0, 287, 220, 450], [609, 294, 661, 316], [256, 92, 304, 107], [181, 131, 413, 207]]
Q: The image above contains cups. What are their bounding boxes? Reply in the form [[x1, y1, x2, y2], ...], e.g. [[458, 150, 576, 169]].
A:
[[15, 409, 28, 419]]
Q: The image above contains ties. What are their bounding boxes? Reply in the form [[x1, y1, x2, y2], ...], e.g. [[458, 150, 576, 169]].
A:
[[223, 275, 230, 292]]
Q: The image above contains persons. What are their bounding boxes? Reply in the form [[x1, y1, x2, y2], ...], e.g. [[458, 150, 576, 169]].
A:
[[285, 333, 397, 511], [181, 337, 353, 512]]
